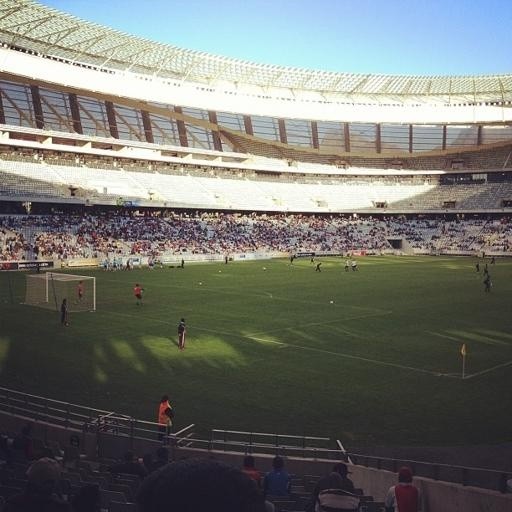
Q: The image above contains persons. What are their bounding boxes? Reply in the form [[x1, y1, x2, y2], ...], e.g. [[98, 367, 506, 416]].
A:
[[2, 426, 62, 511], [59, 298, 69, 327], [385, 467, 421, 512], [110, 446, 290, 510], [2, 144, 512, 273], [157, 394, 176, 433], [177, 317, 187, 352], [75, 280, 86, 303], [313, 463, 354, 500], [482, 274, 491, 294], [133, 283, 145, 306]]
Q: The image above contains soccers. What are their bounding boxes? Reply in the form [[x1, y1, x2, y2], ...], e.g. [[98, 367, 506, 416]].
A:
[[199, 282, 202, 284], [263, 267, 266, 270], [218, 270, 221, 273], [330, 300, 333, 302]]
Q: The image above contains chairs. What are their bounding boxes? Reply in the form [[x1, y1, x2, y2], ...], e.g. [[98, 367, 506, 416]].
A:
[[2, 448, 386, 511]]
